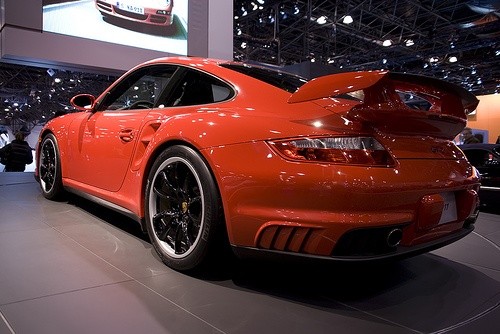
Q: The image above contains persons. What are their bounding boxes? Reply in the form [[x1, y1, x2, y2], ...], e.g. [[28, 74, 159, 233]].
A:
[[0, 131, 33, 172], [0, 125, 13, 172], [462, 127, 480, 144]]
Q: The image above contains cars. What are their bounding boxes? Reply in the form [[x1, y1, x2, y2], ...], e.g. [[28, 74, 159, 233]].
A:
[[95, 0, 175, 26]]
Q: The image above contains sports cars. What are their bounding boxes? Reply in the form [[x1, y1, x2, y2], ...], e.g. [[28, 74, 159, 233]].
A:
[[34, 56, 483, 271], [452, 142, 500, 206]]
